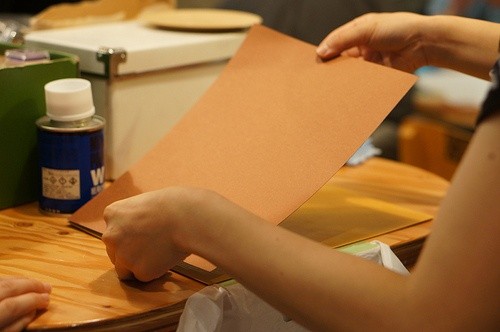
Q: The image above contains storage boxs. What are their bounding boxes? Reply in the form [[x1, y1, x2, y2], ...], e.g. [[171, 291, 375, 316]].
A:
[[25, 18, 248, 180], [0, 41, 78, 211]]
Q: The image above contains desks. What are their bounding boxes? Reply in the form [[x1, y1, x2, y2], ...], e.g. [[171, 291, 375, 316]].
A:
[[0, 152, 452, 332]]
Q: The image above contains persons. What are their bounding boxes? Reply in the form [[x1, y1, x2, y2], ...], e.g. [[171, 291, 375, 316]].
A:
[[0, 272, 52, 332], [101, 11, 500, 332]]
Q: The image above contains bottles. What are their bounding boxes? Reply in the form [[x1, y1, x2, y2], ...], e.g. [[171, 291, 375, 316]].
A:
[[37, 78, 106, 214]]
